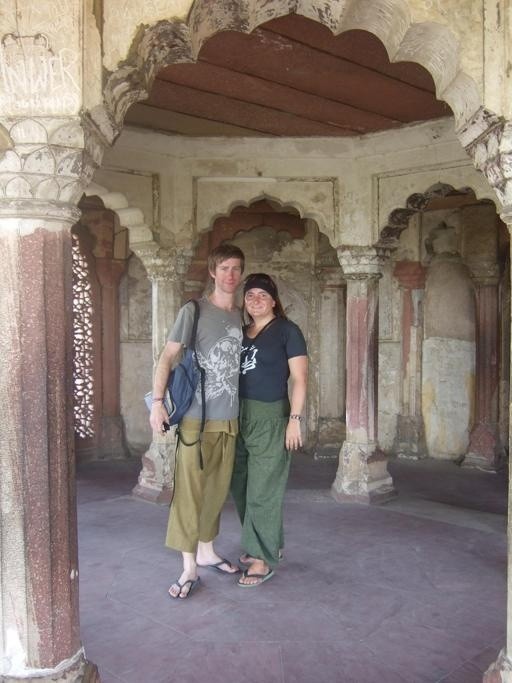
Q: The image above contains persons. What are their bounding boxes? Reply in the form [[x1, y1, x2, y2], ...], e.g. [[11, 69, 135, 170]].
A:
[[148, 245, 251, 601], [230, 271, 312, 588]]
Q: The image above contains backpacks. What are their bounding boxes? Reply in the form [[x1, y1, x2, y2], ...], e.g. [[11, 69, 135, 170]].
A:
[[143, 299, 206, 447]]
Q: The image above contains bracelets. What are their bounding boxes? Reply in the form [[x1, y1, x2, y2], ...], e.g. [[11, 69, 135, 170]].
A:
[[153, 397, 163, 402], [289, 413, 303, 421]]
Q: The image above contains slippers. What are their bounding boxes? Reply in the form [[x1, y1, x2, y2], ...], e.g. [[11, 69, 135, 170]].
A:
[[240, 552, 285, 567], [167, 575, 201, 601], [197, 558, 240, 575], [236, 568, 274, 588]]
